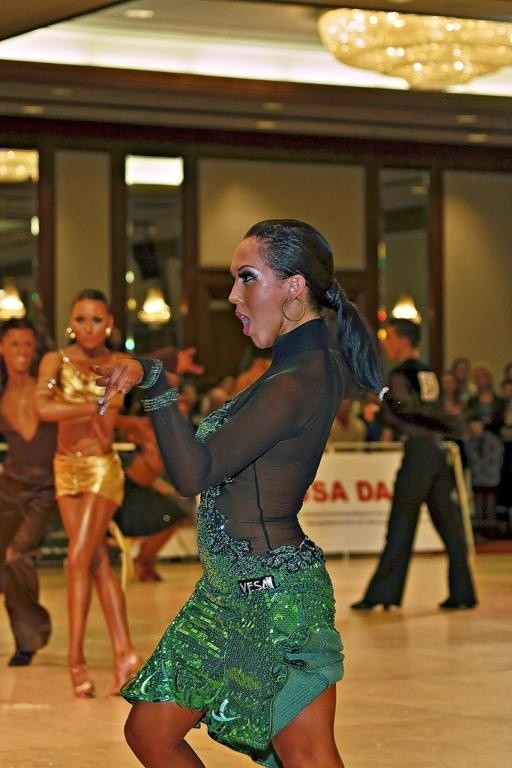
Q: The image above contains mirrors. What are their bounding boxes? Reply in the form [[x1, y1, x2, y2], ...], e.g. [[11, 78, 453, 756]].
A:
[[364, 153, 444, 385], [108, 137, 200, 389], [0, 133, 57, 379]]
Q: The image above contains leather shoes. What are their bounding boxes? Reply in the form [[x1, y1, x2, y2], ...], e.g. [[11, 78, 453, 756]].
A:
[[68, 664, 94, 696], [8, 650, 35, 666], [111, 653, 143, 696]]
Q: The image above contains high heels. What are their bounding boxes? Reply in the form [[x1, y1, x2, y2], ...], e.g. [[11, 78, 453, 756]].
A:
[[441, 596, 475, 609], [350, 597, 391, 610]]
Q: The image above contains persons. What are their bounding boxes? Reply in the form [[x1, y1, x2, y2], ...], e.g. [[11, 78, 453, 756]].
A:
[[91, 217, 395, 767], [30, 344, 511, 582], [1, 317, 58, 665], [350, 316, 484, 611], [34, 288, 142, 699]]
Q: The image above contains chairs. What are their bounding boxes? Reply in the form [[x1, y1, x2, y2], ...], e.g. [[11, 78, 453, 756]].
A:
[[104, 518, 197, 594]]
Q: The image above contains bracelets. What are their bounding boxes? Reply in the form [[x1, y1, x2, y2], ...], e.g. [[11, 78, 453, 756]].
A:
[[136, 358, 163, 389], [141, 386, 182, 413]]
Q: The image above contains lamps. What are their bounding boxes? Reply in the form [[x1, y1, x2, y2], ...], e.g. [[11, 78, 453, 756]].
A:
[[316, 8, 512, 92]]
